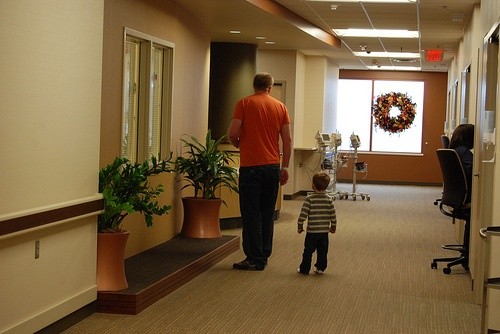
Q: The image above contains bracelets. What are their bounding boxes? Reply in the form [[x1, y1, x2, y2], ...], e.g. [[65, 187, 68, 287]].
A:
[[281, 167, 289, 171]]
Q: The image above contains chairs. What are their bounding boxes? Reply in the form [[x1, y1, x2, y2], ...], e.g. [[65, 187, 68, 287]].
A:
[[430, 134, 473, 276]]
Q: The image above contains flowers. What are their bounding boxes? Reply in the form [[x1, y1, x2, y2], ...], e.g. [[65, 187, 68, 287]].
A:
[[372, 92, 417, 135]]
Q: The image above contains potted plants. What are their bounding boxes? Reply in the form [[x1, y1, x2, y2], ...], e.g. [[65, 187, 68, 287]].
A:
[[172, 127, 241, 239], [95, 151, 174, 292]]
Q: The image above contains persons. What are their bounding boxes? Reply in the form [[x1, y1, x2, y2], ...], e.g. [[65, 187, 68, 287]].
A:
[[229, 72, 292, 270], [297, 173, 337, 276], [448, 123, 475, 267]]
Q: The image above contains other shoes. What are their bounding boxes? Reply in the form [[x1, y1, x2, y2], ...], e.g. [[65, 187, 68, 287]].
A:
[[233, 258, 267, 270], [298, 267, 309, 275], [315, 269, 326, 273]]
